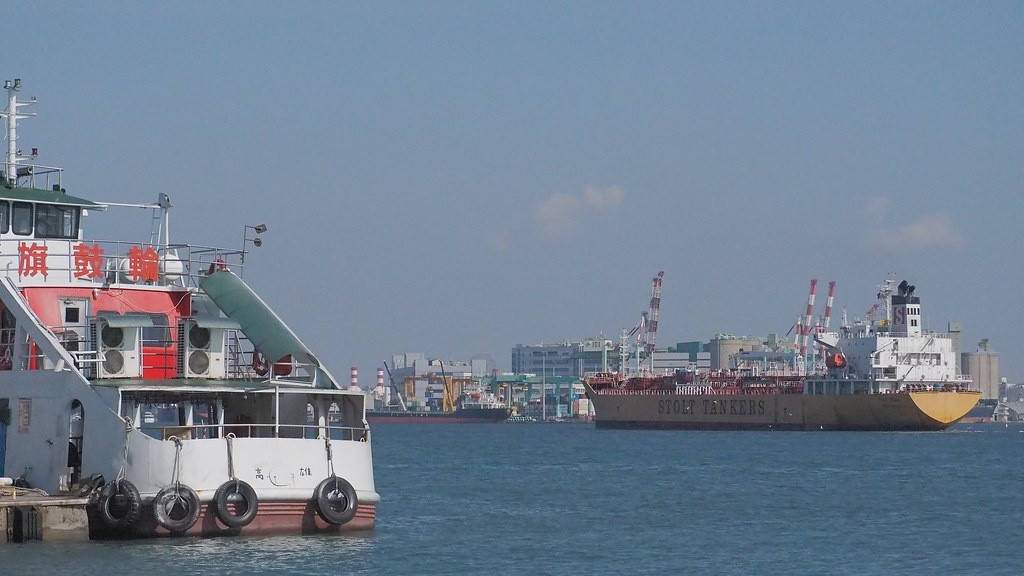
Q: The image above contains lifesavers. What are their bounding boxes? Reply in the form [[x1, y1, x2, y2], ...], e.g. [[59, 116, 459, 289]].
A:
[[96, 478, 142, 533], [252, 349, 269, 376], [150, 482, 202, 534], [312, 475, 360, 526], [212, 478, 260, 529], [209, 258, 226, 275]]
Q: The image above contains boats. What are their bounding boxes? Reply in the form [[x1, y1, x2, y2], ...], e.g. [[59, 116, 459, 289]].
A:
[[826, 353, 846, 369], [366, 358, 514, 423], [578, 269, 985, 431], [0, 76, 382, 537]]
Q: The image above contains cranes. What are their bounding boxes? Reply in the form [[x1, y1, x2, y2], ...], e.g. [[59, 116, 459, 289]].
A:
[[792, 278, 836, 378], [606, 271, 665, 373]]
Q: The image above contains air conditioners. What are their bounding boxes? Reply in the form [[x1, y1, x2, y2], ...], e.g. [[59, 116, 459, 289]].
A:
[[175, 319, 226, 381], [88, 319, 141, 380]]
